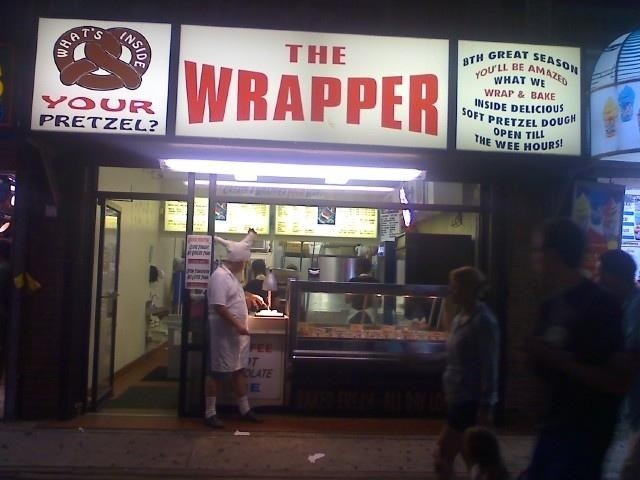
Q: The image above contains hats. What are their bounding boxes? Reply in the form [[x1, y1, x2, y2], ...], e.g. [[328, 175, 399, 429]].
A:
[[214, 227, 258, 263]]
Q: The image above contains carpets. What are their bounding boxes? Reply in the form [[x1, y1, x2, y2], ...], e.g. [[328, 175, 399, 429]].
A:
[[104, 385, 188, 409], [142, 366, 192, 382]]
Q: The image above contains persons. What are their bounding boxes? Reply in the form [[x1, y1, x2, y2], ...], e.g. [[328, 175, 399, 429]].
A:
[[596, 246, 639, 479], [519, 220, 625, 478], [342, 258, 384, 326], [203, 243, 266, 430], [433, 266, 502, 480], [243, 258, 280, 309], [462, 422, 509, 479]]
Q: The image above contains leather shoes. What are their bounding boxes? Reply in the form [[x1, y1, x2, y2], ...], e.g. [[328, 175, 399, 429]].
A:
[[204, 415, 224, 427], [240, 411, 265, 422]]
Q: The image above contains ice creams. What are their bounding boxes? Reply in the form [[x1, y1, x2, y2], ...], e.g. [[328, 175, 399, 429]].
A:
[[602, 85, 635, 138]]
